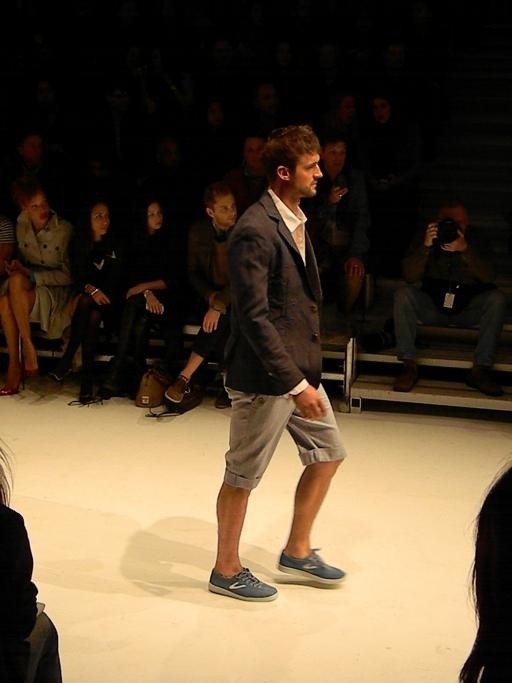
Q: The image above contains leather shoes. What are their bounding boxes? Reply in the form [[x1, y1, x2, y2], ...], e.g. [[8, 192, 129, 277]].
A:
[[465, 368, 505, 397], [394, 361, 418, 391]]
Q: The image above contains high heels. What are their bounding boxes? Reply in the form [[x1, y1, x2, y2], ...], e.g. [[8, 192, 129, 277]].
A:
[[95, 377, 114, 401], [47, 365, 72, 389], [77, 377, 94, 403], [1, 364, 25, 396], [24, 355, 39, 378]]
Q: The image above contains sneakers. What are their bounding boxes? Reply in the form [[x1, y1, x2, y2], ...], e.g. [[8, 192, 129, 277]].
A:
[[277, 550, 346, 584], [214, 390, 229, 410], [166, 376, 187, 403], [208, 568, 278, 602]]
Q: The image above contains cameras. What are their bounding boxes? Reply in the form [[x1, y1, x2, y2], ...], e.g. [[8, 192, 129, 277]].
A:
[[437, 217, 458, 244]]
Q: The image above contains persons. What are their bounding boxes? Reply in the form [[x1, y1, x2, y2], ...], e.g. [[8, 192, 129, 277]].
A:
[[0, 2, 511, 421], [457, 463, 509, 681], [1, 440, 64, 680], [207, 126, 349, 602]]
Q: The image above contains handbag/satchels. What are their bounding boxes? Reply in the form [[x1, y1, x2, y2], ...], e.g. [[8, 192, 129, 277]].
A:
[[135, 368, 168, 408], [166, 383, 203, 415]]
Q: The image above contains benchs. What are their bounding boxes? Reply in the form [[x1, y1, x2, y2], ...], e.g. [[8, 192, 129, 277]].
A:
[[347, 272, 512, 418], [0, 306, 347, 392]]
